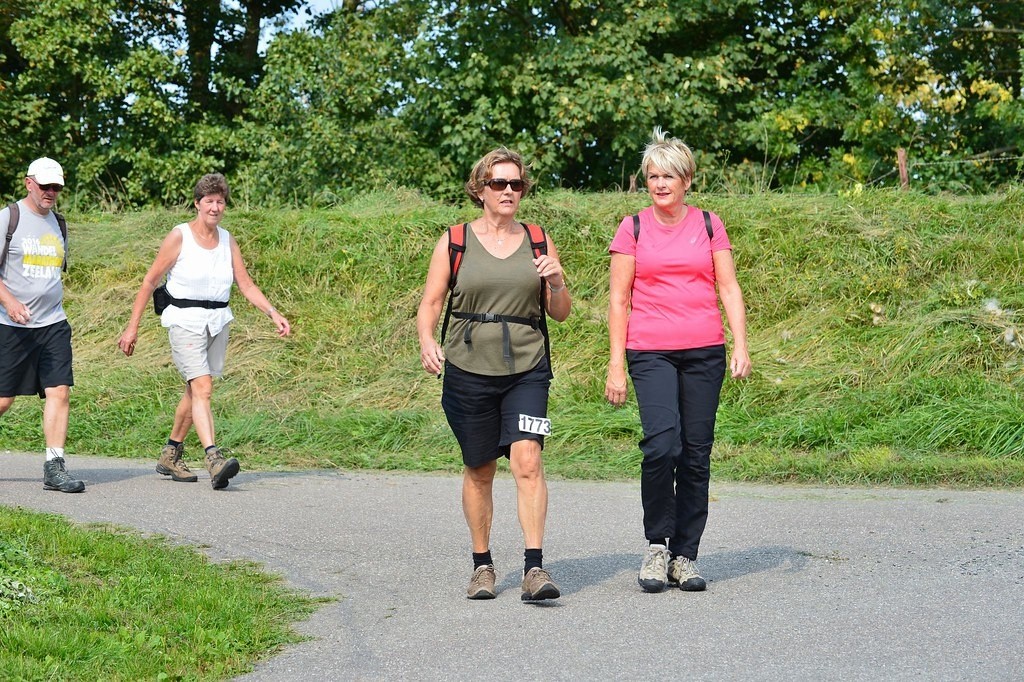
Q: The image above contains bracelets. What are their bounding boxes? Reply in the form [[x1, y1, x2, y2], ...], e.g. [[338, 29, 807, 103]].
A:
[[548, 279, 565, 292], [266, 307, 276, 317]]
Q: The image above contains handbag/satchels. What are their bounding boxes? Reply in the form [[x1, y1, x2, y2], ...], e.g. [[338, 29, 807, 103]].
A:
[[153, 284, 170, 315]]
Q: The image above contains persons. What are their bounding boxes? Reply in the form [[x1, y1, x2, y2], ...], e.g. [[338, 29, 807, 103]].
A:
[[119, 174, 290, 490], [0, 158, 85, 495], [417, 148, 572, 601], [605, 128, 752, 591]]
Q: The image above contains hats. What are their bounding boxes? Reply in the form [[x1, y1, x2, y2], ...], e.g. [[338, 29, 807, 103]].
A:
[[26, 156, 65, 185]]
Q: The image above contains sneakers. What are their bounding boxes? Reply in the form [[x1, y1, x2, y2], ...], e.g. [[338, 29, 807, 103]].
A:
[[520, 566, 560, 601], [156, 444, 198, 482], [637, 542, 672, 592], [467, 564, 501, 599], [42, 447, 85, 493], [204, 446, 240, 490], [668, 555, 707, 591]]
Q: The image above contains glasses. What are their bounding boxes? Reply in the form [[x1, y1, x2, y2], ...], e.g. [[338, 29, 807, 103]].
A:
[[30, 178, 63, 192], [484, 178, 525, 191]]
